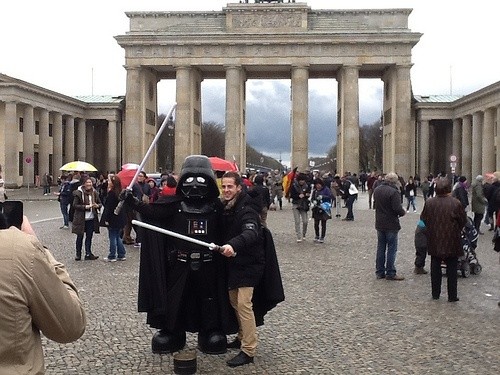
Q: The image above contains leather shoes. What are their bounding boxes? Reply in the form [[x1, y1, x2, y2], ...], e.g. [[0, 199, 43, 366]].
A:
[[226, 337, 240, 349], [226, 351, 253, 368]]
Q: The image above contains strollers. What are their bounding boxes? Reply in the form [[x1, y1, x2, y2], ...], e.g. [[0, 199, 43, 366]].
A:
[[440, 216, 483, 278]]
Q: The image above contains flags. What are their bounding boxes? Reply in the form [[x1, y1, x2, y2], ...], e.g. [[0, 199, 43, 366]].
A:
[[283, 168, 297, 193]]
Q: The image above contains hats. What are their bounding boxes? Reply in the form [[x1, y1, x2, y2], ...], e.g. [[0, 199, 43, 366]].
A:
[[459, 176, 467, 183]]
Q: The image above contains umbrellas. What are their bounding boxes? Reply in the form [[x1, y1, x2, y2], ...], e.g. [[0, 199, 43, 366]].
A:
[[59, 161, 98, 171], [208, 157, 236, 171]]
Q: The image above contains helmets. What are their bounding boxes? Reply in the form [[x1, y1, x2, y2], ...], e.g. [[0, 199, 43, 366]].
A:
[[176, 154, 220, 207]]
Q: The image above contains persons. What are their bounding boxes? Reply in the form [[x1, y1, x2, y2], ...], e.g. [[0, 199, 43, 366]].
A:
[[327, 170, 386, 220], [54, 171, 115, 229], [243, 170, 284, 210], [43, 173, 53, 195], [122, 171, 177, 247], [118, 155, 239, 354], [374, 172, 406, 280], [72, 177, 102, 260], [220, 171, 265, 366], [0, 216, 87, 375], [0, 179, 6, 202], [414, 176, 468, 302], [453, 170, 500, 234], [100, 175, 126, 262], [311, 178, 332, 242], [290, 173, 311, 242], [396, 176, 436, 213]]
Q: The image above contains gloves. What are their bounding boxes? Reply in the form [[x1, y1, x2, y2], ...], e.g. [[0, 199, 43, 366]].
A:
[[118, 189, 139, 208]]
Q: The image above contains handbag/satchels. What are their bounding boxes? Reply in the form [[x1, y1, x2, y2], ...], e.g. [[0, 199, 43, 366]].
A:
[[142, 194, 149, 203], [304, 199, 310, 211], [345, 180, 358, 195], [410, 189, 414, 197]]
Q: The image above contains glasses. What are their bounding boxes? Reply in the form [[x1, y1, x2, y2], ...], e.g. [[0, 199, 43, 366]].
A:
[[138, 175, 143, 177]]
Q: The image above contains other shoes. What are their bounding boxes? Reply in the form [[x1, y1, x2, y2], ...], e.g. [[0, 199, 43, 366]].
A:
[[297, 237, 305, 242], [85, 254, 99, 260], [386, 275, 404, 280], [75, 254, 81, 260], [448, 298, 459, 301], [121, 235, 140, 248], [313, 236, 324, 242], [342, 217, 354, 221], [478, 231, 484, 235], [117, 256, 126, 260], [104, 258, 117, 262], [414, 265, 427, 274], [377, 275, 386, 279]]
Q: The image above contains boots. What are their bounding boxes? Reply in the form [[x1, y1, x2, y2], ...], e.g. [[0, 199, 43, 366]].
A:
[[489, 223, 494, 231], [494, 226, 497, 232]]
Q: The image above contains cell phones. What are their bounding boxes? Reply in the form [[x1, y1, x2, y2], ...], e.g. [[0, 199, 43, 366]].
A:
[[0, 200, 23, 230]]
